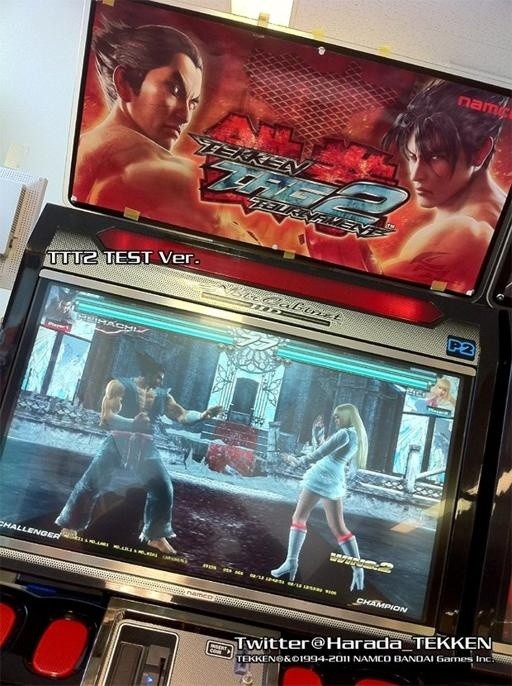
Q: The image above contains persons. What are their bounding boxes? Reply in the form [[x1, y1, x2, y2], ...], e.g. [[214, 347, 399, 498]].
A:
[[366, 76, 507, 298], [55, 352, 226, 556], [424, 377, 456, 416], [72, 9, 222, 237], [269, 403, 369, 594]]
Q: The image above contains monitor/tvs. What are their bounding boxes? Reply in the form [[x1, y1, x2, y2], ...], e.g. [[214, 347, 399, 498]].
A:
[[0, 270, 479, 636]]
